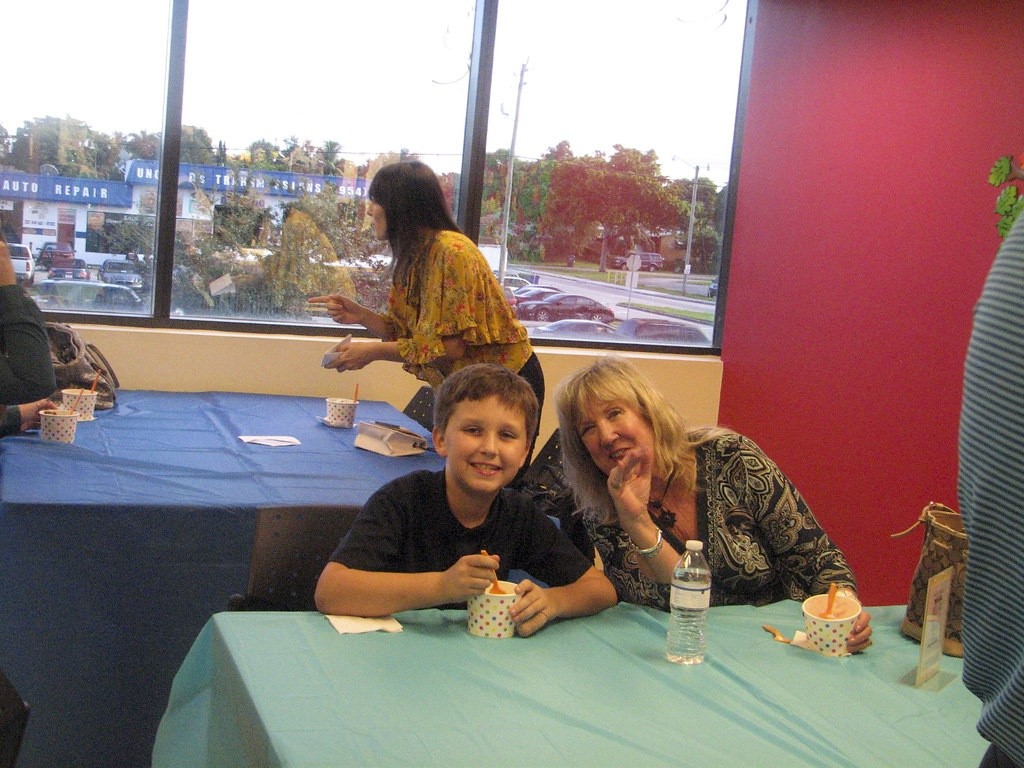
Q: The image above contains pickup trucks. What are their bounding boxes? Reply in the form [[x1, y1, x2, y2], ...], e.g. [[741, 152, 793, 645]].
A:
[[8, 243, 37, 286]]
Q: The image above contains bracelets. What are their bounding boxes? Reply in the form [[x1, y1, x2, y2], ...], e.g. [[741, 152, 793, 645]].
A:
[[629, 525, 665, 558]]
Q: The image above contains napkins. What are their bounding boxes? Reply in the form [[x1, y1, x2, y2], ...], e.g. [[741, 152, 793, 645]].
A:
[[325, 612, 403, 634], [239, 435, 301, 447]]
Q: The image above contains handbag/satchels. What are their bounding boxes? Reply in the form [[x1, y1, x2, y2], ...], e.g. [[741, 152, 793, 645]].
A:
[[891, 502, 965, 658], [45, 321, 120, 410], [353, 420, 430, 456]]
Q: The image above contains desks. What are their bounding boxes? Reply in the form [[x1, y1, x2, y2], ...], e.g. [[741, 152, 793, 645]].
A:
[[0, 391, 447, 767], [152, 604, 991, 768]]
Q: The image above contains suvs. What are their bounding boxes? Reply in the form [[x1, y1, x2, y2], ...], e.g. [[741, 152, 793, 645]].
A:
[[613, 252, 664, 272]]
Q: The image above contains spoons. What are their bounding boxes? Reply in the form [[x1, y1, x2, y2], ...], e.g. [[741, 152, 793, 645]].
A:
[[818, 583, 837, 619], [481, 550, 507, 594], [763, 624, 793, 642]]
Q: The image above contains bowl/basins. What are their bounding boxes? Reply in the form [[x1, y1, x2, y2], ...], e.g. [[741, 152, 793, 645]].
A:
[[326, 398, 359, 427], [468, 581, 526, 639], [802, 594, 862, 656], [39, 410, 79, 443], [61, 389, 98, 420]]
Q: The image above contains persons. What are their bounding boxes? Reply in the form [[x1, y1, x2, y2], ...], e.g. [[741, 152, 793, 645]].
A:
[[311, 362, 617, 636], [551, 353, 874, 654], [308, 160, 545, 477], [957, 197, 1022, 768], [0, 242, 62, 436]]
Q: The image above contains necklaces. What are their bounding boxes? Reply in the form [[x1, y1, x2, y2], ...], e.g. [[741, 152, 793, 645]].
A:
[[649, 463, 677, 528]]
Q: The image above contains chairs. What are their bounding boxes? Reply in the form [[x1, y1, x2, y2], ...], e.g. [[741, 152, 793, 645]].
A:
[[227, 505, 362, 611]]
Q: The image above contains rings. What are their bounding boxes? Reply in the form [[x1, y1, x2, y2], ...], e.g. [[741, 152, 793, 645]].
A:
[[609, 479, 622, 489]]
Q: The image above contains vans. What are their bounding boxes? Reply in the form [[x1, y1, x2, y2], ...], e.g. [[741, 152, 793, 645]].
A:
[[616, 318, 709, 344]]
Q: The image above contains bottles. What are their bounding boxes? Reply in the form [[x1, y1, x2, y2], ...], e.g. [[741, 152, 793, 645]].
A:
[[664, 540, 712, 665]]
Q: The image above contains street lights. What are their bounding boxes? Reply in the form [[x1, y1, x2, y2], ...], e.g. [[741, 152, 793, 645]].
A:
[[496, 58, 549, 287], [671, 150, 711, 295]]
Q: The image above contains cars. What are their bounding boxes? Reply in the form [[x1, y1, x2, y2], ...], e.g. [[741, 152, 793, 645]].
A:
[[492, 270, 565, 306], [515, 294, 614, 323], [97, 259, 144, 293], [36, 241, 75, 267], [530, 318, 628, 339], [47, 258, 91, 281], [708, 274, 719, 298]]
